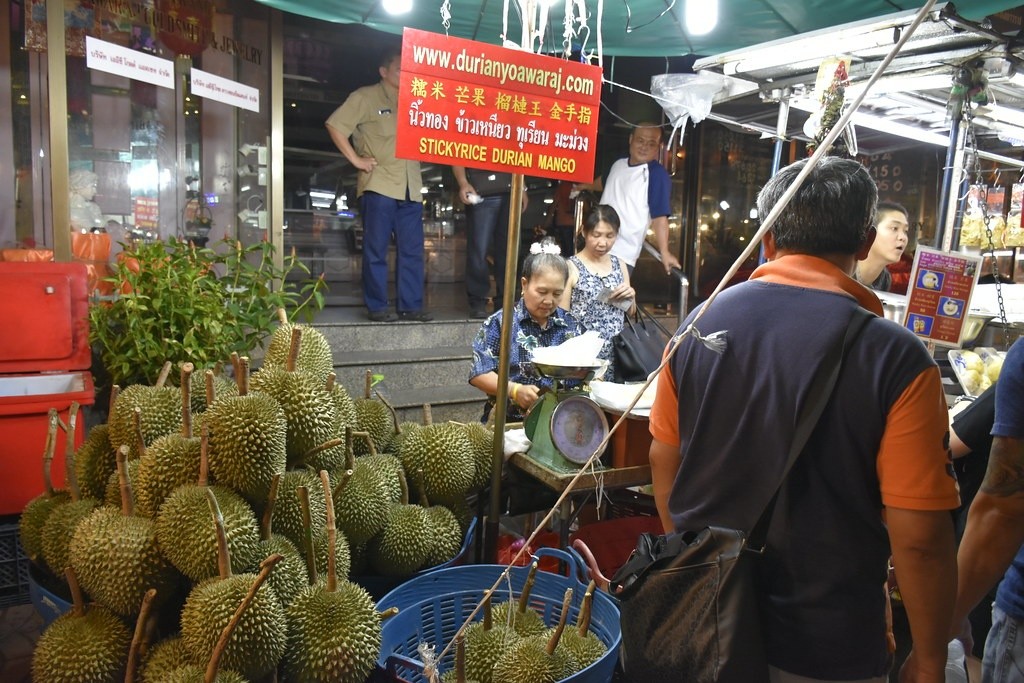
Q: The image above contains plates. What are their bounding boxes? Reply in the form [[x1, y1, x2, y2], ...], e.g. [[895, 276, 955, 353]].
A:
[[948, 347, 1007, 399]]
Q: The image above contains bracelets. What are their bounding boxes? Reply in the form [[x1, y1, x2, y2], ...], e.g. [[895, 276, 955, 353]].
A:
[[509, 383, 522, 401]]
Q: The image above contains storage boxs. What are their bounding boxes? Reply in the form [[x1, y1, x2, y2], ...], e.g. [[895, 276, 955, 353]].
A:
[[0, 261, 88, 608]]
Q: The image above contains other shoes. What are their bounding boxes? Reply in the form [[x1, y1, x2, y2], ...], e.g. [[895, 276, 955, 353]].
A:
[[468, 303, 488, 317], [399, 310, 433, 321], [369, 310, 399, 321]]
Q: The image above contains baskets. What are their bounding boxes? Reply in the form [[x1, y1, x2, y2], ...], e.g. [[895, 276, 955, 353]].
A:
[[351, 515, 663, 683]]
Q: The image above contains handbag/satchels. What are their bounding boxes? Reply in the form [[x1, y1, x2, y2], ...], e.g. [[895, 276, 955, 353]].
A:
[[608, 523, 771, 683], [612, 307, 669, 383]]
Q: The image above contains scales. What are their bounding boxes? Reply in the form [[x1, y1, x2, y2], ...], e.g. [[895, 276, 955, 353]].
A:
[[522, 359, 610, 474]]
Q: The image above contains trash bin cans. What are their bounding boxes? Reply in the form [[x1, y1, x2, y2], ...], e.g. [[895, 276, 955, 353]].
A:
[[1, 260, 97, 518]]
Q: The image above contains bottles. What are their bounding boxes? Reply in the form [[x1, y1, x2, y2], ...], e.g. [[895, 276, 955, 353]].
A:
[[991, 601, 1005, 626], [945, 638, 967, 683]]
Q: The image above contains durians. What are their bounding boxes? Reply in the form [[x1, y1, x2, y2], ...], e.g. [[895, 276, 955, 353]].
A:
[[441, 600, 609, 683], [20, 321, 497, 683]]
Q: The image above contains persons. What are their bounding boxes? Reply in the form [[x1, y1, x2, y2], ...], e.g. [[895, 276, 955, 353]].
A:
[[957, 335, 1024, 683], [71, 170, 104, 230], [545, 181, 574, 258], [326, 50, 434, 321], [648, 156, 961, 683], [851, 201, 910, 291], [453, 165, 528, 318], [560, 204, 637, 382], [469, 252, 589, 423], [572, 121, 681, 279]]
